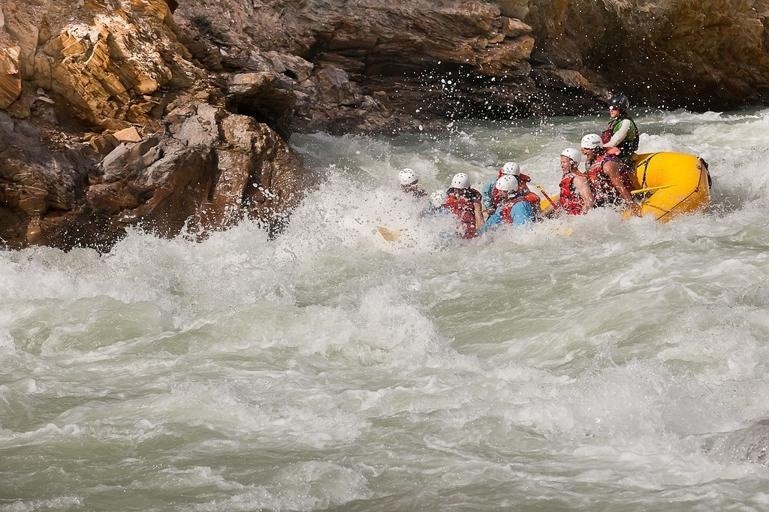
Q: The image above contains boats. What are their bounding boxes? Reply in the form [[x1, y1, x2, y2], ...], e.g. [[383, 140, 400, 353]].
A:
[[540, 151, 713, 227]]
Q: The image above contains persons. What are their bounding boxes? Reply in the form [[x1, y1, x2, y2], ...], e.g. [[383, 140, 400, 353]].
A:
[[397, 94, 640, 244]]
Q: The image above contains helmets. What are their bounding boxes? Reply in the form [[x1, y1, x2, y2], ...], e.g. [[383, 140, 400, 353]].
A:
[[580, 134, 603, 150], [399, 168, 417, 186], [500, 162, 520, 176], [560, 147, 581, 166], [495, 175, 518, 191], [451, 172, 471, 190], [608, 94, 629, 109]]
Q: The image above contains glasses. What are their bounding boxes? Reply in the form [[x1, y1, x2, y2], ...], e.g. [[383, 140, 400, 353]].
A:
[[403, 179, 418, 187]]
